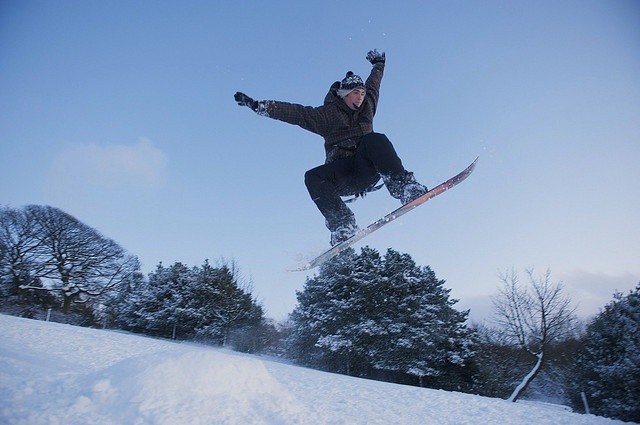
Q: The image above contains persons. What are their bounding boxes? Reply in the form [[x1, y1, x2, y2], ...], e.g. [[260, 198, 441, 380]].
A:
[[234, 50, 428, 247]]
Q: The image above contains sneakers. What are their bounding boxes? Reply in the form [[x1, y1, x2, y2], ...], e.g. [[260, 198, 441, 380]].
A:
[[400, 182, 428, 205], [331, 217, 360, 246]]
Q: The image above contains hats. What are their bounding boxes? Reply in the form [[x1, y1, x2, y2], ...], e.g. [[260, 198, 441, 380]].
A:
[[337, 71, 367, 99]]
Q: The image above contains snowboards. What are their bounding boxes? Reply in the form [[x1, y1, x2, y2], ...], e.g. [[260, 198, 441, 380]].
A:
[[287, 157, 480, 271]]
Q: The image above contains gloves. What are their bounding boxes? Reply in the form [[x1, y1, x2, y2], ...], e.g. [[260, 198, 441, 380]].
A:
[[234, 92, 258, 111], [366, 49, 385, 64]]
